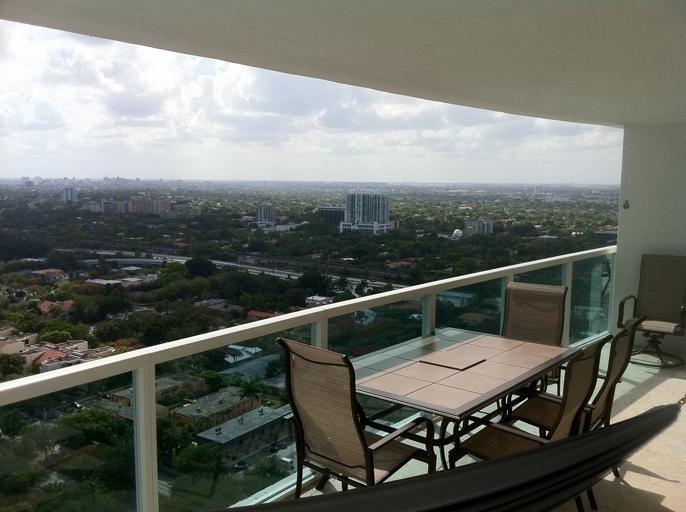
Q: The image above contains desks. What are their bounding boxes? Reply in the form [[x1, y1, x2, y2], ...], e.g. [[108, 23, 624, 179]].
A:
[[339, 328, 584, 471], [339, 335, 584, 471]]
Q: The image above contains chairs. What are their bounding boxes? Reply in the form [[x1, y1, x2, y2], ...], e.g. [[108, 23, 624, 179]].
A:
[[620, 254, 686, 368], [277, 336, 436, 499], [498, 316, 645, 480], [497, 285, 569, 415], [278, 331, 436, 499], [450, 331, 614, 511]]
